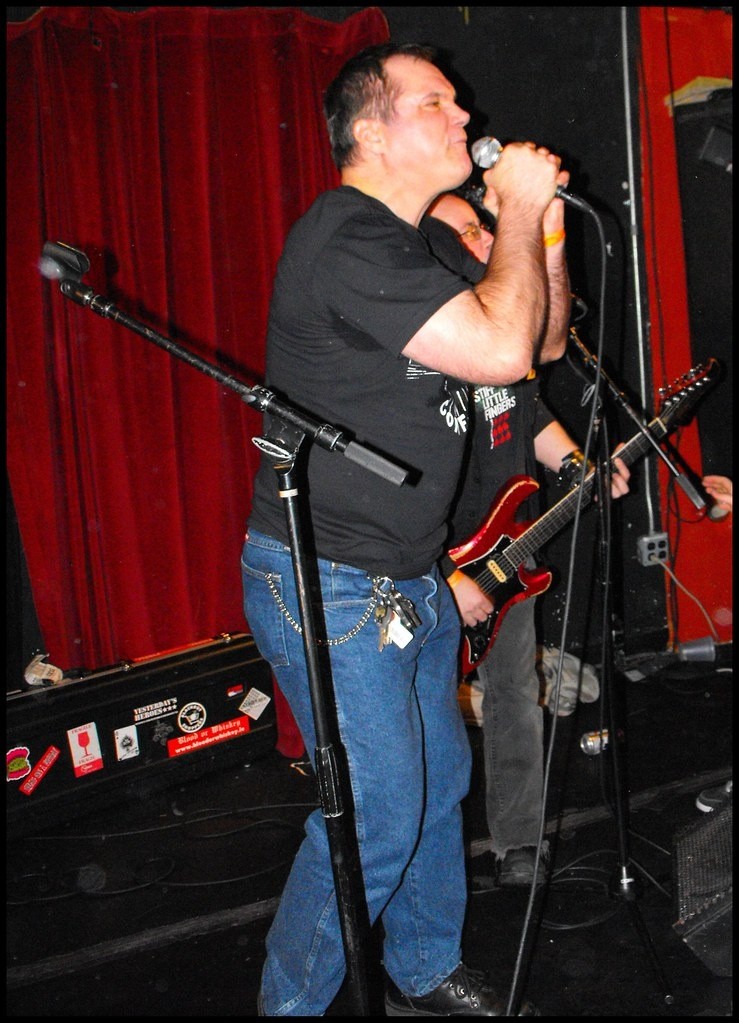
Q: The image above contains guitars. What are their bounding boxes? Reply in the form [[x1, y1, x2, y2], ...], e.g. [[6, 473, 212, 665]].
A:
[[448, 355, 722, 676]]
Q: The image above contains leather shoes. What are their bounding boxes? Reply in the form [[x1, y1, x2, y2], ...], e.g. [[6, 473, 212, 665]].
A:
[[385, 962, 541, 1017]]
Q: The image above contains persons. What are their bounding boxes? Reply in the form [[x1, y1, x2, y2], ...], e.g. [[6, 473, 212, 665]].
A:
[[240, 36, 570, 1017], [425, 192, 630, 887]]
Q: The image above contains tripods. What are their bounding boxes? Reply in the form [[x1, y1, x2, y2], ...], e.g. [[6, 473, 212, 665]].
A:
[[544, 321, 708, 1006]]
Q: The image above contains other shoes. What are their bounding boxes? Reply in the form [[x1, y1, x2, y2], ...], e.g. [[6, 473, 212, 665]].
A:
[[493, 846, 550, 887]]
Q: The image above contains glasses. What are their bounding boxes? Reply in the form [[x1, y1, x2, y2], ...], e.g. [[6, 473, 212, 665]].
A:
[[458, 222, 490, 243]]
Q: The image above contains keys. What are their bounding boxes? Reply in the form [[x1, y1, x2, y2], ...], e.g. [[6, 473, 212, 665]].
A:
[[375, 590, 422, 653]]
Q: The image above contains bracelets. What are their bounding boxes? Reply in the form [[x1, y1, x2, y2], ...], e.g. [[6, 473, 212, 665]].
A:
[[543, 229, 566, 247]]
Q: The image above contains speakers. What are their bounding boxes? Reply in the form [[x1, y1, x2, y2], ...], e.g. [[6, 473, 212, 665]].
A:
[[671, 798, 733, 979], [672, 89, 735, 479]]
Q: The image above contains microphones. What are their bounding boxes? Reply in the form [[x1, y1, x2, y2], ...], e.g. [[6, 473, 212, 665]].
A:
[[473, 136, 593, 214]]
